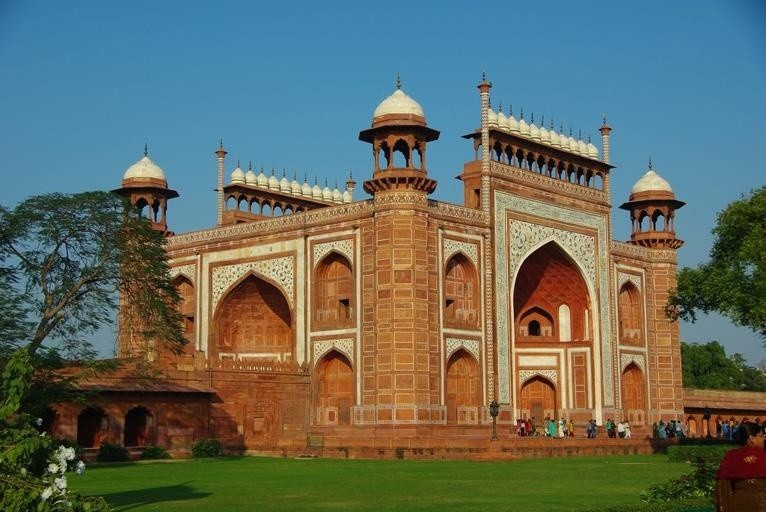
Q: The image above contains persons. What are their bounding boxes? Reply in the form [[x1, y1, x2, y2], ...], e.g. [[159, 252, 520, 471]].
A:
[[716, 419, 740, 441], [623, 419, 632, 438], [607, 418, 612, 438], [658, 418, 689, 439], [611, 419, 617, 438], [717, 420, 766, 478], [516, 414, 599, 437], [618, 421, 625, 438]]
